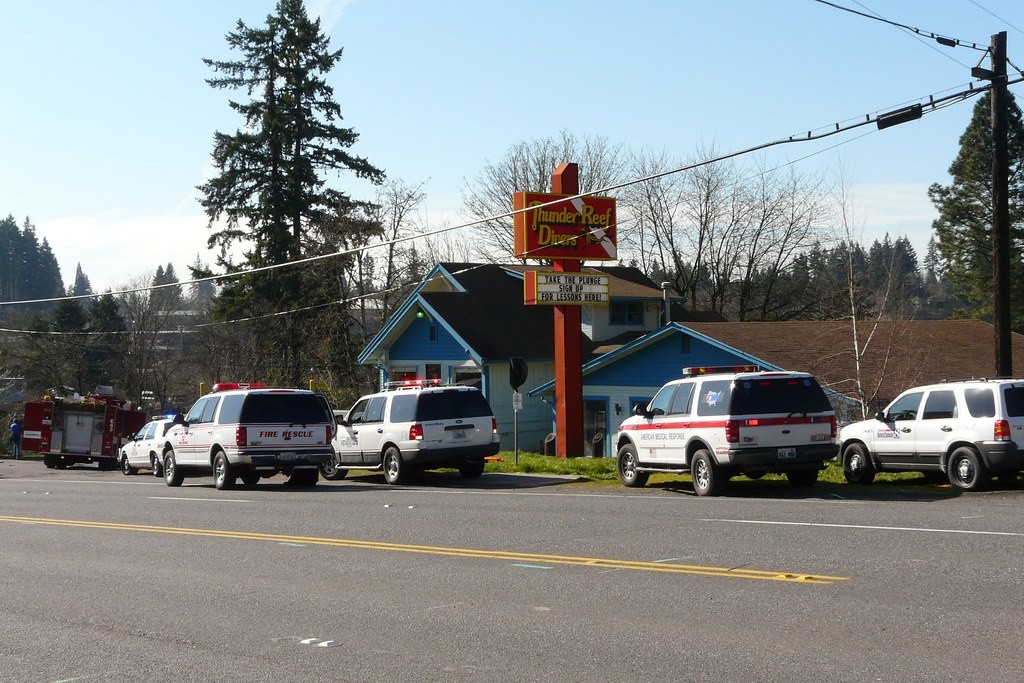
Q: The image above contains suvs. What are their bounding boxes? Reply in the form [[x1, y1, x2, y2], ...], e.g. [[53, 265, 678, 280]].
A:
[[320, 378, 500, 485], [158, 382, 337, 490], [616, 364, 840, 496], [119, 415, 177, 477], [837, 377, 1024, 491]]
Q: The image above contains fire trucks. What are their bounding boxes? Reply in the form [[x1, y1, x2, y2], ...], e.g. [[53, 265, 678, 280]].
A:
[[21, 394, 148, 471]]
